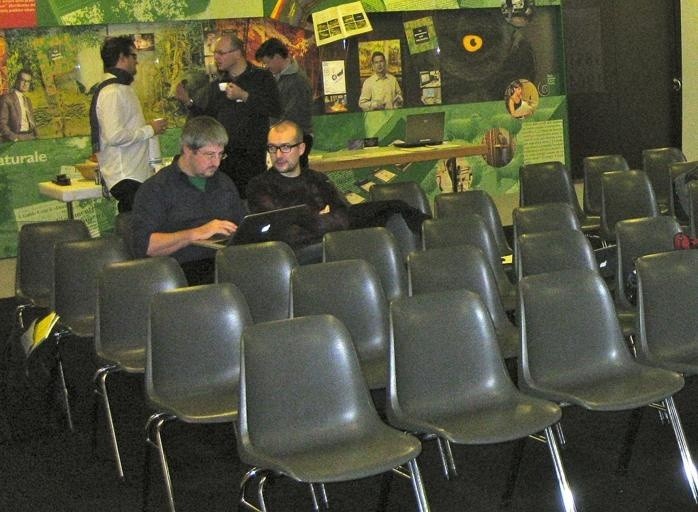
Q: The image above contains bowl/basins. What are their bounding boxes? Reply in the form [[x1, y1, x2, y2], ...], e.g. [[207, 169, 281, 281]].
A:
[[74, 164, 99, 180]]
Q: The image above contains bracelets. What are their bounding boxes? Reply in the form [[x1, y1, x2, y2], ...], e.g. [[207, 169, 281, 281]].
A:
[[184, 99, 193, 107]]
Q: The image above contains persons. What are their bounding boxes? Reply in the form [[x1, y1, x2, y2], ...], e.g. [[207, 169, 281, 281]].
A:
[[0, 69, 39, 142], [357, 51, 404, 112], [507, 81, 536, 118], [246, 120, 354, 248], [255, 38, 314, 170], [125, 116, 252, 285], [176, 33, 283, 200], [88, 35, 169, 214]]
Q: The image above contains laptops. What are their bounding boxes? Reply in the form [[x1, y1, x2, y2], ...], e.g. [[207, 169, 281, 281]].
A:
[[205, 204, 305, 246], [393, 111, 445, 147]]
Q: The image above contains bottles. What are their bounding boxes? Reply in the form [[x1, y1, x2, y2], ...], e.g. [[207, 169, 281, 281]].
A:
[[95, 175, 100, 185], [149, 136, 161, 162]]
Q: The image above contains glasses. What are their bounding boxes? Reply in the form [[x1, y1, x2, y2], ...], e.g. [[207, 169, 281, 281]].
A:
[[185, 148, 228, 160], [213, 48, 242, 56], [121, 52, 139, 61], [265, 142, 306, 153], [18, 78, 32, 83]]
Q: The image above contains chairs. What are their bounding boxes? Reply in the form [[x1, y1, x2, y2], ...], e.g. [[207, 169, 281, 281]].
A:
[[5, 141, 697, 512]]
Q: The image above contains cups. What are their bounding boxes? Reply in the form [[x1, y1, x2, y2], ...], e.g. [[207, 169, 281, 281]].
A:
[[154, 118, 166, 130], [218, 83, 228, 92]]
